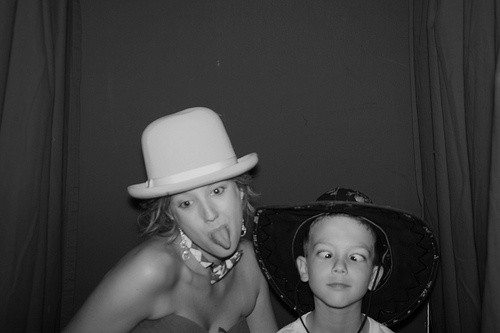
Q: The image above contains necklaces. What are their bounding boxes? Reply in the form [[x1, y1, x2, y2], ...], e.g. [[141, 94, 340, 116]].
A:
[[177, 224, 243, 284]]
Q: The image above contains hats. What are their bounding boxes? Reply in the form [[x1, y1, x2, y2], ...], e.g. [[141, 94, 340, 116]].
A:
[[252, 188, 439, 328], [127, 107, 259, 198]]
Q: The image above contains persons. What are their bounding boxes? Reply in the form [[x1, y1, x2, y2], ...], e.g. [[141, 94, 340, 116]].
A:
[[275, 188, 396, 333], [62, 107, 277, 333]]
